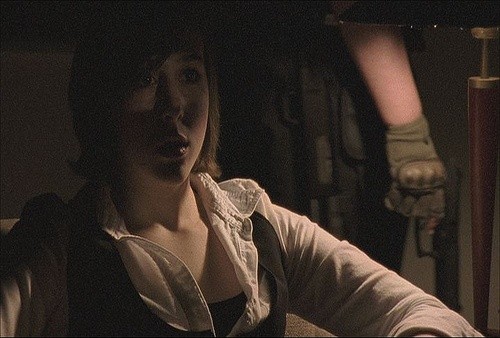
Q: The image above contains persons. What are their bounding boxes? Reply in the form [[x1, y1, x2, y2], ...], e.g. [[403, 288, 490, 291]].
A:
[[0, 0, 463, 338], [332, 0, 446, 258]]
